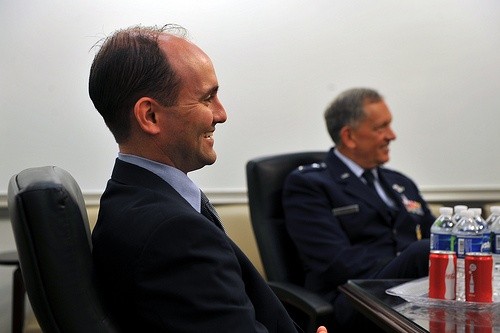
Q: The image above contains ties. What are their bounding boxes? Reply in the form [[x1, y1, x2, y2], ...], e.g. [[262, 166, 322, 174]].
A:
[[365, 169, 381, 201], [199, 189, 225, 233]]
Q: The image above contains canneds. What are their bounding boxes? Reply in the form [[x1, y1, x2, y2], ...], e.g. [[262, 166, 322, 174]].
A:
[[464, 252, 493, 302], [428, 250, 458, 300], [429, 306, 493, 333]]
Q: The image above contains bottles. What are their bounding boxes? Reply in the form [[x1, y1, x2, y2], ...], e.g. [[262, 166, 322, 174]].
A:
[[429, 204, 500, 303]]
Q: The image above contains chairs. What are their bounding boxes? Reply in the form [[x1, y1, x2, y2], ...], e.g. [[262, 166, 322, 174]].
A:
[[246, 153, 334, 333], [7, 166, 117, 333]]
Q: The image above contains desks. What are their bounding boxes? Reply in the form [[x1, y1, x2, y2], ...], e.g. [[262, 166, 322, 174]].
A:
[[338, 278, 429, 333]]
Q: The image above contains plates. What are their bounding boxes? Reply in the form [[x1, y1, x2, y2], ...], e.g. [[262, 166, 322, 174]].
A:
[[385, 276, 500, 313]]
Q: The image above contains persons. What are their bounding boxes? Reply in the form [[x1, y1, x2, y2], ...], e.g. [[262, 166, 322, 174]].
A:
[[88, 26, 327, 333], [284, 86, 437, 299]]
